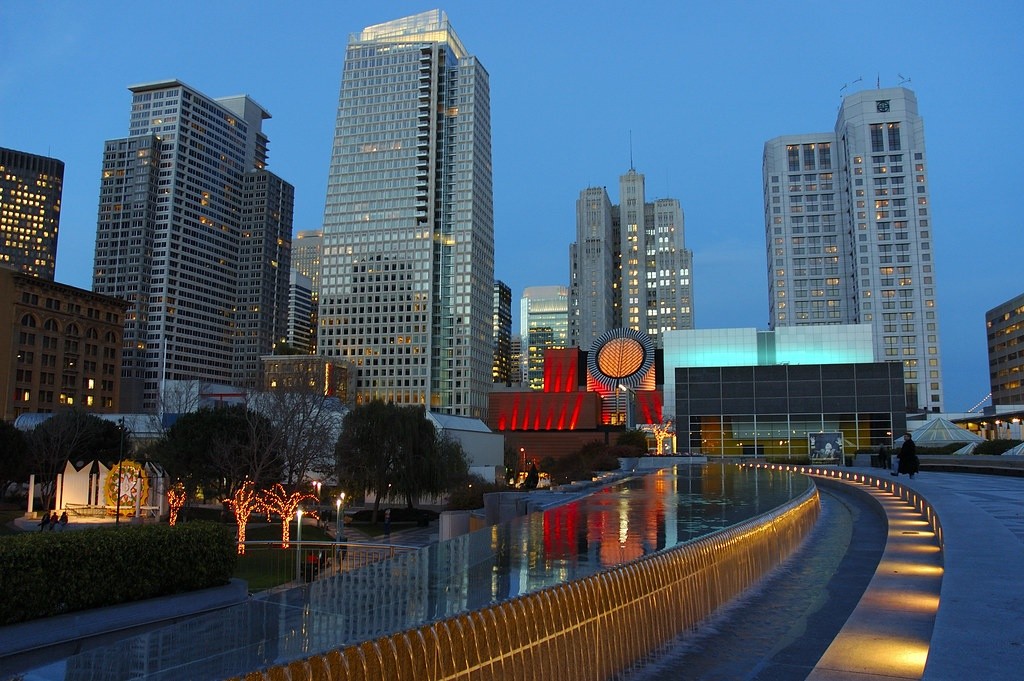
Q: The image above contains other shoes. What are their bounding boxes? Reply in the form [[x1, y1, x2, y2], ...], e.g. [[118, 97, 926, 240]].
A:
[[890, 472, 898, 476], [38, 524, 40, 526], [40, 529, 43, 532]]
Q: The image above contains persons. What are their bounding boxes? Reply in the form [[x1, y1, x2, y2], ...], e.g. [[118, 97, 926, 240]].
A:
[[891, 433, 920, 479], [58, 512, 68, 526], [37, 510, 50, 531], [337, 537, 347, 573], [300, 581, 312, 615], [383, 509, 390, 544], [505, 469, 514, 486], [48, 512, 59, 531], [304, 552, 317, 582], [879, 444, 892, 469]]
